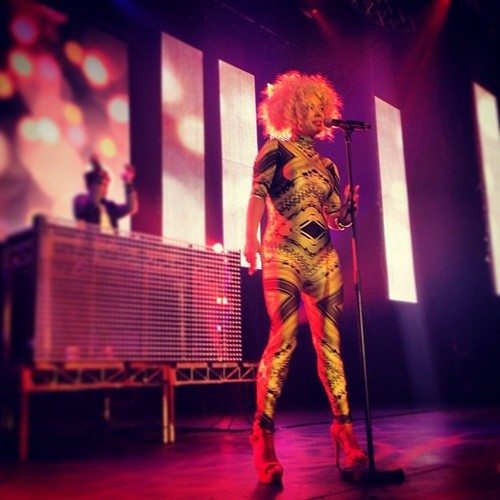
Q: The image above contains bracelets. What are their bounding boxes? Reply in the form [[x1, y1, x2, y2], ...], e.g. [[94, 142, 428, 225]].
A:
[[338, 222, 353, 228]]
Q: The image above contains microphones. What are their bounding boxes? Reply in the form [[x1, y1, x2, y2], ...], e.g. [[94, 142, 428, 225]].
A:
[[324, 117, 371, 130]]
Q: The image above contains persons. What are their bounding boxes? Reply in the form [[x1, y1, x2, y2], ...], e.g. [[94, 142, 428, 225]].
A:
[[243, 70, 370, 485], [73, 156, 139, 235]]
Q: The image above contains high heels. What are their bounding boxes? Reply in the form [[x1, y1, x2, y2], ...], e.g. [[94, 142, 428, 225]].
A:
[[248, 429, 283, 485], [329, 417, 369, 468]]
